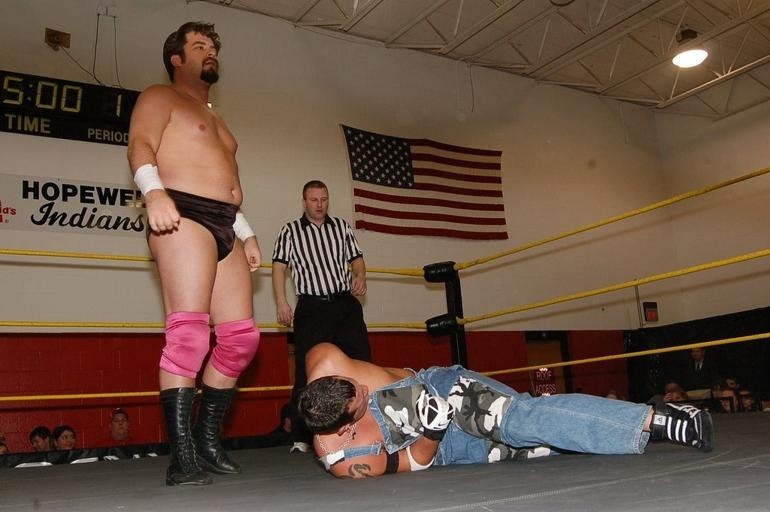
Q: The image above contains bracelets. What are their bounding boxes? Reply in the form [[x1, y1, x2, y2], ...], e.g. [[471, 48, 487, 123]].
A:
[[233, 212, 256, 246], [135, 165, 165, 196]]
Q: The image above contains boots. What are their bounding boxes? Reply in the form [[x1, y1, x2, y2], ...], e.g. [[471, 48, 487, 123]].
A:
[[158, 383, 216, 489], [190, 382, 243, 476]]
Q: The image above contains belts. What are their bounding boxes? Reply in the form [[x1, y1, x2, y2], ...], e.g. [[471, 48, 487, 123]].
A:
[[297, 289, 355, 304]]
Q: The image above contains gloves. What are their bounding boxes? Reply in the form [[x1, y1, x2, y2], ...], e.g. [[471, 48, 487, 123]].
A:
[[414, 389, 455, 431]]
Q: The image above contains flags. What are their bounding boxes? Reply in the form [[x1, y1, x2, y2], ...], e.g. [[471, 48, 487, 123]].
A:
[[339, 122, 509, 241]]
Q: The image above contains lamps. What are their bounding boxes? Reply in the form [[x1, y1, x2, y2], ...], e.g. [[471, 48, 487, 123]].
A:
[[672, 25, 709, 69]]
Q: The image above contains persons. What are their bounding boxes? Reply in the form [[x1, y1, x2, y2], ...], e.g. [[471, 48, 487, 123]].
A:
[[274, 179, 368, 454], [297, 341, 715, 478], [125, 21, 263, 487], [606, 341, 770, 413], [0, 408, 128, 463], [267, 398, 293, 447]]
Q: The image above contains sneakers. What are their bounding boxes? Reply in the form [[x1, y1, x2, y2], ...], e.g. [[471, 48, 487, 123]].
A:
[[648, 400, 716, 450]]
